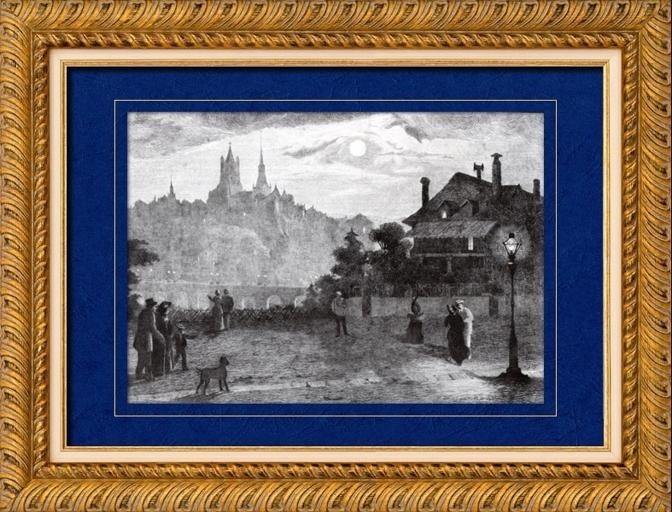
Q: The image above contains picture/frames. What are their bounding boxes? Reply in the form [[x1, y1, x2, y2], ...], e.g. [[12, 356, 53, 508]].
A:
[[0, 0, 672, 506]]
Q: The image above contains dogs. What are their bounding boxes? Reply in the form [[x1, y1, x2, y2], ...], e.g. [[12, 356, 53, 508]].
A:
[[194, 356, 231, 396]]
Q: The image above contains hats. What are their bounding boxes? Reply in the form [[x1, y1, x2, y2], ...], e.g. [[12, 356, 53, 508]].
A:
[[156, 300, 173, 311], [175, 322, 185, 330], [454, 299, 465, 307], [144, 295, 158, 309]]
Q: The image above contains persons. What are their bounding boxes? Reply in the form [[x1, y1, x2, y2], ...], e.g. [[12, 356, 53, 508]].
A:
[[331, 290, 350, 338], [170, 324, 200, 371], [220, 289, 234, 330], [152, 300, 171, 377], [443, 305, 471, 365], [133, 296, 165, 382], [208, 289, 224, 335], [447, 299, 474, 362]]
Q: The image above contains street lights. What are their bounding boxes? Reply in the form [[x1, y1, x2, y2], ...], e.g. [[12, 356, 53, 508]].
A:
[[502, 234, 524, 377]]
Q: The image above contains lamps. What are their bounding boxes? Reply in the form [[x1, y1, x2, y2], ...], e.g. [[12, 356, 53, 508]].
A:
[[493, 230, 531, 390]]
